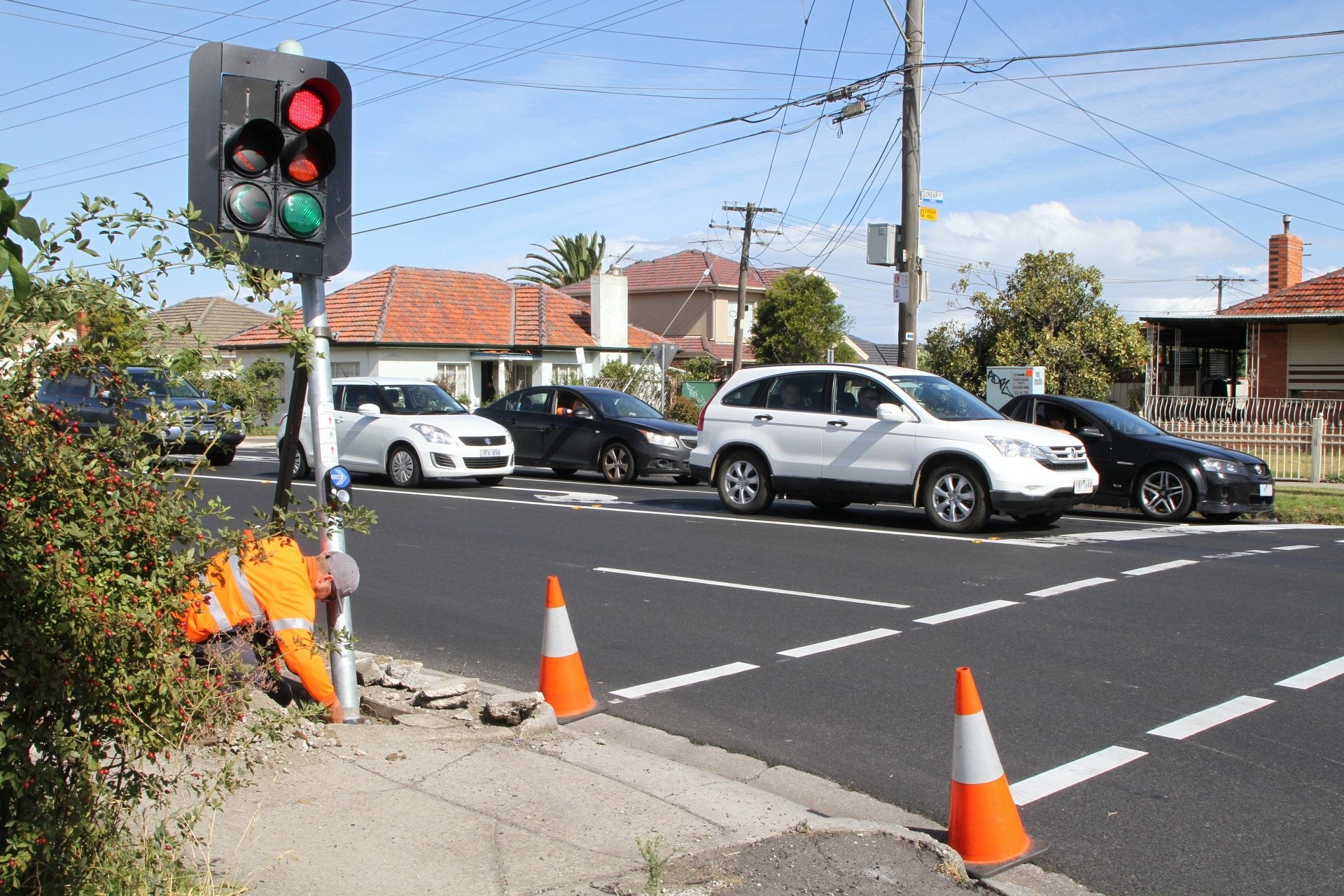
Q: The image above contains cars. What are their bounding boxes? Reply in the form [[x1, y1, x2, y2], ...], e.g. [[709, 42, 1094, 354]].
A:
[[997, 393, 1276, 522], [472, 384, 701, 486], [276, 376, 515, 490]]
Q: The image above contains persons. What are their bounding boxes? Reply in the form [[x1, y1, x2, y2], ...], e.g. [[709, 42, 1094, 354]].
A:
[[484, 380, 496, 402], [353, 390, 367, 408], [882, 386, 904, 409], [779, 381, 802, 410], [846, 386, 878, 416], [1044, 406, 1081, 439], [556, 395, 582, 416], [171, 531, 360, 723]]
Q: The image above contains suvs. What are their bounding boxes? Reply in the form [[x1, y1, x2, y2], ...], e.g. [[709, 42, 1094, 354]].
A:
[[688, 363, 1101, 533], [35, 363, 248, 466]]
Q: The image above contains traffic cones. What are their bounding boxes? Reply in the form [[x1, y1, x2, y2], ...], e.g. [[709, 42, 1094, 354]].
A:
[[948, 666, 1053, 883], [537, 575, 605, 725]]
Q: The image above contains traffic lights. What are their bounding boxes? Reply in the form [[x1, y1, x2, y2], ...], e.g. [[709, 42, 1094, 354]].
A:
[[217, 73, 340, 244]]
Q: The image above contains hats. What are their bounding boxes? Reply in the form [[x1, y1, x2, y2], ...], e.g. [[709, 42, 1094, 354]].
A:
[[325, 552, 360, 613]]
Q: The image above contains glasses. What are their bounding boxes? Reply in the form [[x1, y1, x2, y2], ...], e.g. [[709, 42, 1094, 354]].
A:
[[861, 395, 879, 400], [326, 582, 336, 603], [1054, 419, 1064, 423]]
